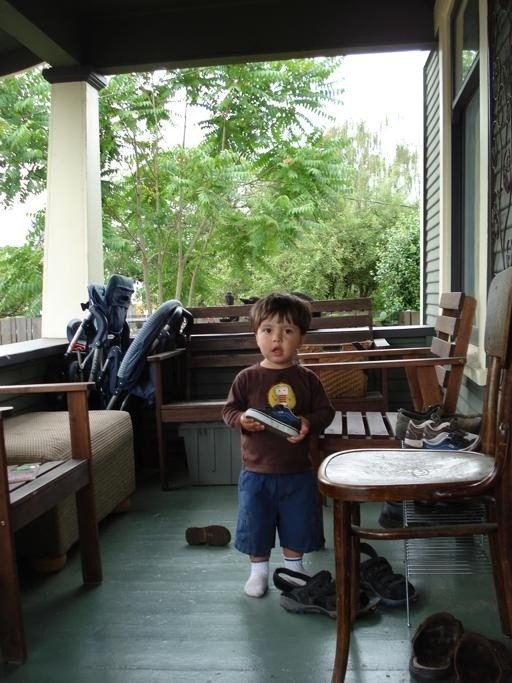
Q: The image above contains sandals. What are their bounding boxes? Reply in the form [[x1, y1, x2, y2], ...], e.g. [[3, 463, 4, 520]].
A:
[[409, 612, 512, 683], [273, 543, 417, 620]]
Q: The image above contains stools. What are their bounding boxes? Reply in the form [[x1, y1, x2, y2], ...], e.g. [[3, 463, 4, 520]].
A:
[[2, 411, 136, 572]]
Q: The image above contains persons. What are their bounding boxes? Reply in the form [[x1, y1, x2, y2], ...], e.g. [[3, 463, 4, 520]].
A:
[[220, 291, 336, 598]]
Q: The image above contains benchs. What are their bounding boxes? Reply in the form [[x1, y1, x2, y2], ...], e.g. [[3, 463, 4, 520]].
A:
[[146, 295, 374, 492]]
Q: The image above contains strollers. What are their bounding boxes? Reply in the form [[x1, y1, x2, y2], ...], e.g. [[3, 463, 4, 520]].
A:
[[56, 274, 194, 425]]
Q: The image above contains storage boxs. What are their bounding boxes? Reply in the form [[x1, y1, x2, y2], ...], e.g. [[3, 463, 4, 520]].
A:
[[177, 420, 240, 487]]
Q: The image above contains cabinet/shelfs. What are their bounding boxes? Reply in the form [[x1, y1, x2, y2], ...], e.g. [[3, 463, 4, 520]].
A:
[[399, 440, 495, 628]]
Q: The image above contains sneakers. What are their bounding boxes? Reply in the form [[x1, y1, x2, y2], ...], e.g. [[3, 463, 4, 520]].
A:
[[378, 500, 486, 529], [185, 525, 231, 547], [395, 404, 481, 452], [245, 405, 301, 440]]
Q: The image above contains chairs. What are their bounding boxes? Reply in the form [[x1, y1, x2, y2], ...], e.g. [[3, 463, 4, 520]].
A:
[[0, 381, 99, 666], [314, 264, 512, 681], [300, 290, 477, 551]]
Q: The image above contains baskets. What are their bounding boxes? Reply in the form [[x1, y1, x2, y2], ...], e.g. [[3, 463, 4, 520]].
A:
[[296, 340, 374, 399]]
